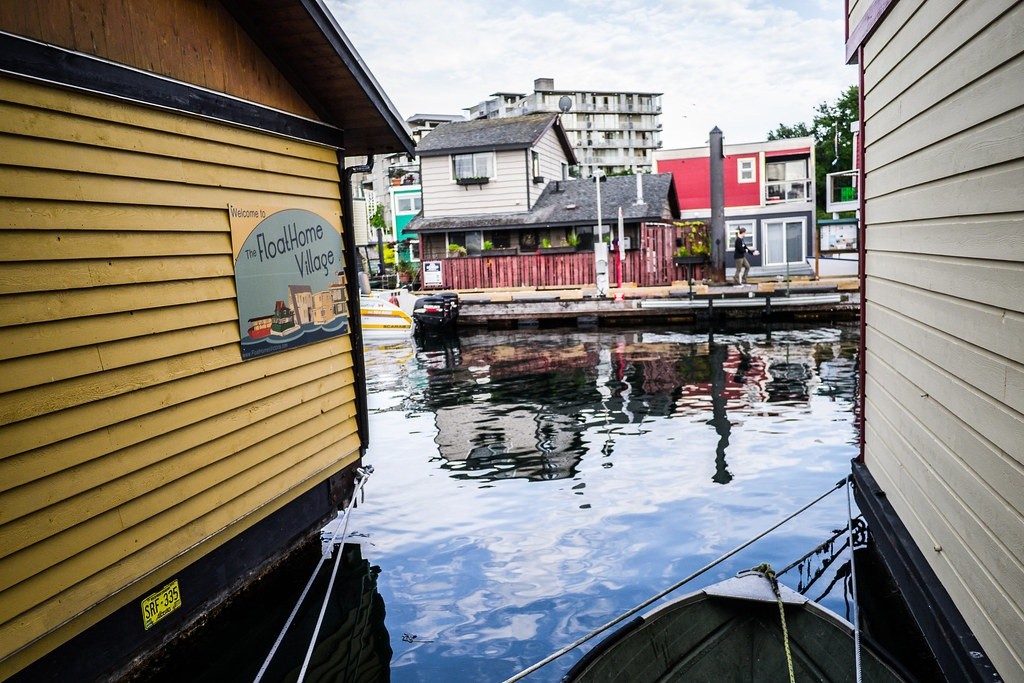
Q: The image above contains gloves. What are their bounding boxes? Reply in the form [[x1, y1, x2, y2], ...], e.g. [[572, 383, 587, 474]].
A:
[[752, 250, 760, 256]]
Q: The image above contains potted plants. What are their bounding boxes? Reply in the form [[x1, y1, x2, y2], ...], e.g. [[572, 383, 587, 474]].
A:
[[448, 244, 467, 259], [672, 242, 709, 265], [480, 240, 517, 257], [455, 177, 490, 186], [393, 260, 416, 284], [387, 168, 407, 185], [538, 226, 582, 254], [404, 177, 416, 185]]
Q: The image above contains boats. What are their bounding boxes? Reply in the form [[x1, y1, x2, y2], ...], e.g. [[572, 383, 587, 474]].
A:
[[359, 288, 420, 346], [556, 565, 918, 683]]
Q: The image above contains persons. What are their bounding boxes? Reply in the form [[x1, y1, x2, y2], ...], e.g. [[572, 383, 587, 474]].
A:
[[734, 228, 760, 284]]
